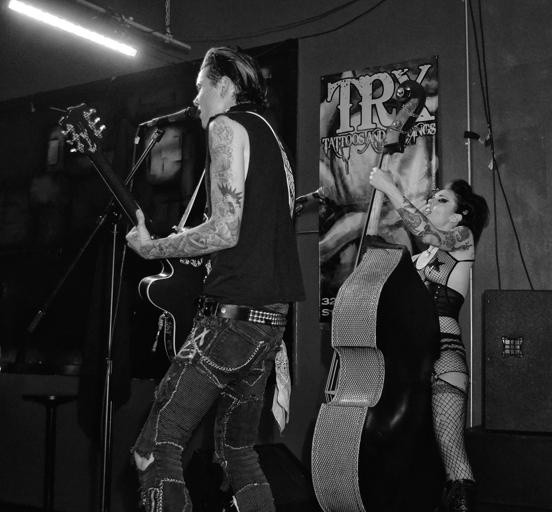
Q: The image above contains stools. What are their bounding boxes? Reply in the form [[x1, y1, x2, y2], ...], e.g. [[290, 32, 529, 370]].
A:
[[24, 392, 81, 511]]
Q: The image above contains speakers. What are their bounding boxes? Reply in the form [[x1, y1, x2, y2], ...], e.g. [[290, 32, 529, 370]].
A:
[[481, 289, 552, 435]]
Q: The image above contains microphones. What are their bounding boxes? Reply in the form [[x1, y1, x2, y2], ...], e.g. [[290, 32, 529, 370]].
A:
[[146, 106, 195, 128], [295, 186, 331, 205]]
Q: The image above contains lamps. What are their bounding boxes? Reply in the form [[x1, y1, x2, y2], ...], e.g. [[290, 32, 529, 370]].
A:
[[10, 0, 193, 68]]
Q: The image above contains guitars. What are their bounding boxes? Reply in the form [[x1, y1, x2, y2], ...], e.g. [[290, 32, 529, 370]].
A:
[[57, 99, 203, 364]]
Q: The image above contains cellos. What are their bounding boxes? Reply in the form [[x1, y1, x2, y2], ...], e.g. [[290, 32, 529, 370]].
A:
[[309, 81, 441, 512]]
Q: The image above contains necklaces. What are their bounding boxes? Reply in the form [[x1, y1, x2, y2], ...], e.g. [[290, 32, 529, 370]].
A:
[[425, 246, 435, 258]]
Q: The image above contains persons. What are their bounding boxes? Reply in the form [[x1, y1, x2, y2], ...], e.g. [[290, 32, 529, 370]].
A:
[[368, 164, 491, 510], [125, 46, 304, 511]]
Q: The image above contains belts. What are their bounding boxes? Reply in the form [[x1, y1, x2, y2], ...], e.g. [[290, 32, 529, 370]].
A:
[[198, 298, 288, 328]]
